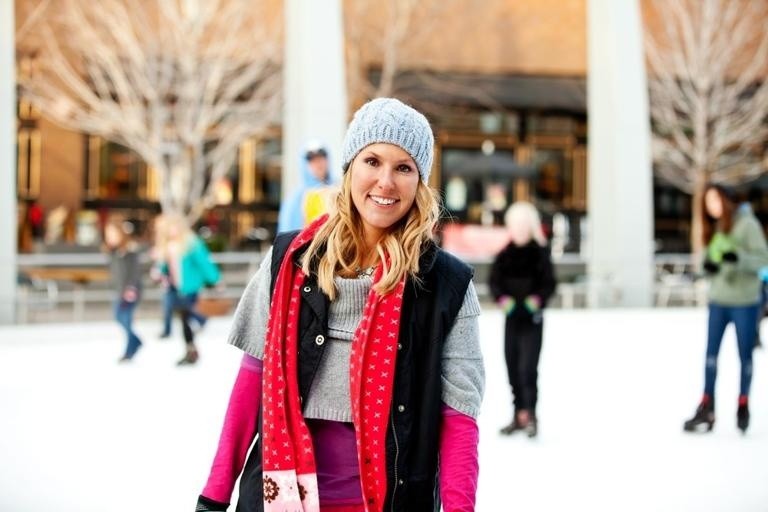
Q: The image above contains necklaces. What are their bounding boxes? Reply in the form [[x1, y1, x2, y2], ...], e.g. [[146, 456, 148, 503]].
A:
[[347, 252, 383, 280]]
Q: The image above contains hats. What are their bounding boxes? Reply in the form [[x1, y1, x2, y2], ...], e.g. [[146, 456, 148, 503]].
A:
[[340, 97, 436, 185]]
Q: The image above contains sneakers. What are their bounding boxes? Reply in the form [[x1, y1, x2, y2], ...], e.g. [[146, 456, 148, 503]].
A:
[[498, 418, 538, 439], [178, 348, 199, 366]]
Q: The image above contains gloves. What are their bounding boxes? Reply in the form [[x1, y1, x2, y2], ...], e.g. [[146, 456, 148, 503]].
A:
[[498, 294, 540, 317], [703, 250, 740, 274]]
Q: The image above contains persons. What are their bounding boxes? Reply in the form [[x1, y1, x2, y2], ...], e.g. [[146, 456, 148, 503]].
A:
[[486, 197, 561, 439], [105, 216, 144, 365], [149, 212, 223, 366], [300, 139, 340, 232], [680, 182, 767, 433], [196, 95, 487, 512], [159, 214, 208, 341], [22, 200, 276, 293]]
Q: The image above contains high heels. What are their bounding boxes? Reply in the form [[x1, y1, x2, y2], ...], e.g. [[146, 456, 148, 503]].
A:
[[735, 403, 752, 433], [683, 404, 718, 435]]
